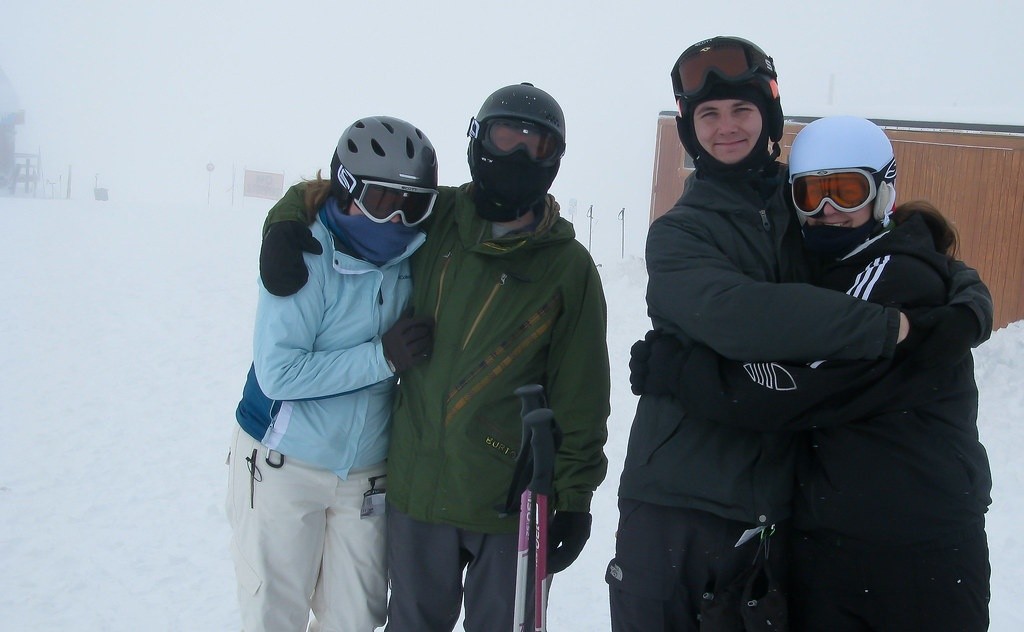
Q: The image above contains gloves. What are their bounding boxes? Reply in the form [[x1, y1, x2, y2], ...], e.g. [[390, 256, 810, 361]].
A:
[[547, 510, 592, 575], [382, 307, 433, 375], [629, 331, 690, 397], [909, 302, 980, 378], [260, 223, 323, 296]]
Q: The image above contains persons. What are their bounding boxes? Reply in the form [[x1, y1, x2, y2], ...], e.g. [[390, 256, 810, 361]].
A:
[[600, 36, 993, 632], [260, 81, 608, 631], [228, 115, 439, 632], [628, 113, 990, 632]]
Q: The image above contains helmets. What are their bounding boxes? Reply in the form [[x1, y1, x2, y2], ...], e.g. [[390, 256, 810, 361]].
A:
[[469, 84, 567, 220], [672, 38, 784, 184], [331, 120, 440, 227], [790, 115, 898, 226]]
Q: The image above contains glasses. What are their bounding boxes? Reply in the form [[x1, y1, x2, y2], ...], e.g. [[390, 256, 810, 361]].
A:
[[790, 162, 881, 218], [480, 118, 564, 165], [671, 39, 762, 96], [352, 180, 439, 226]]
